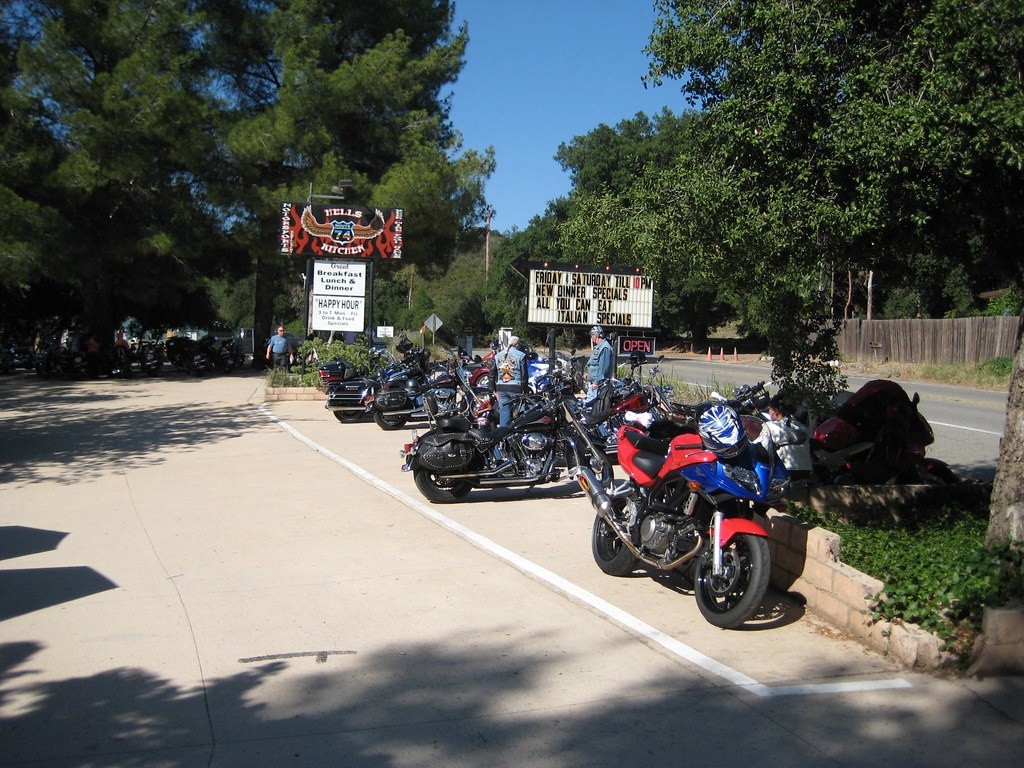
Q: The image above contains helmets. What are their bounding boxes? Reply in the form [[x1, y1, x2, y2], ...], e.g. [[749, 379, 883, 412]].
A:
[[700, 404, 749, 461], [590, 326, 604, 334]]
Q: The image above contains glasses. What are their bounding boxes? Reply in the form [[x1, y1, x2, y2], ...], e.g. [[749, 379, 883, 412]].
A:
[[279, 330, 285, 333]]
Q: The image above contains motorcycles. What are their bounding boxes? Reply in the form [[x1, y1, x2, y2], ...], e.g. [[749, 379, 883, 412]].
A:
[[316, 324, 960, 629], [34, 324, 245, 381]]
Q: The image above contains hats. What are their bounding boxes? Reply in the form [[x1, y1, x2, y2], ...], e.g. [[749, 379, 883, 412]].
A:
[[508, 336, 521, 347]]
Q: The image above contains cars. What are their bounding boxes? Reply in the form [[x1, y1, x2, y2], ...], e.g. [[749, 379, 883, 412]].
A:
[[251, 332, 304, 369]]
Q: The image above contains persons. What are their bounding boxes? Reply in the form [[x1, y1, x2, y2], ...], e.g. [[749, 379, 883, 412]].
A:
[[115, 332, 129, 348], [489, 335, 528, 441], [748, 393, 813, 479], [580, 326, 614, 414], [85, 331, 100, 380], [267, 325, 294, 370]]
